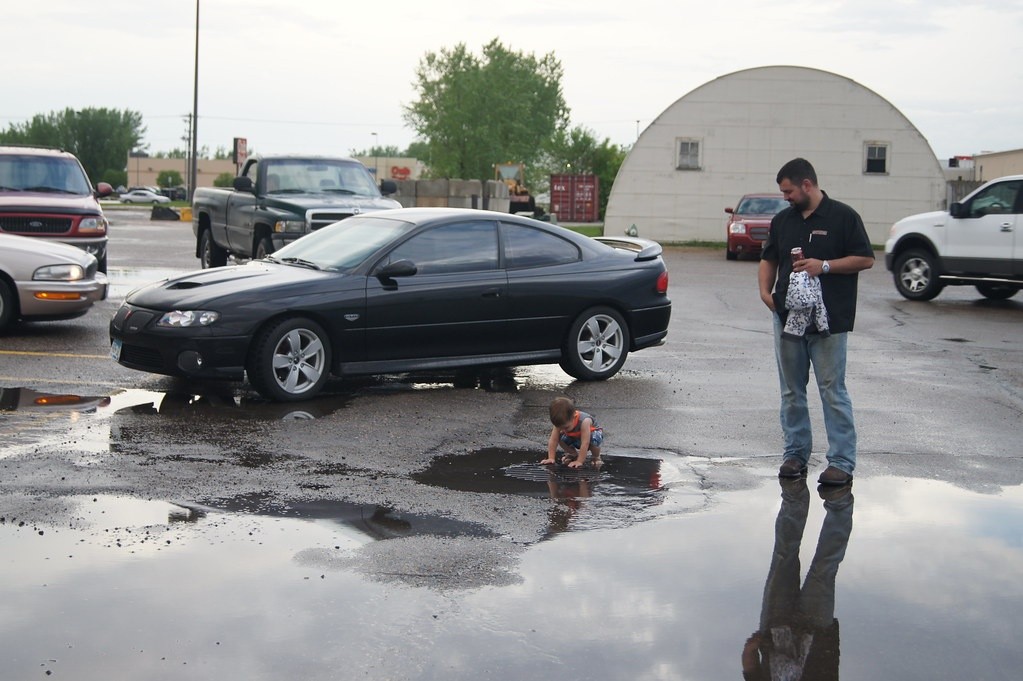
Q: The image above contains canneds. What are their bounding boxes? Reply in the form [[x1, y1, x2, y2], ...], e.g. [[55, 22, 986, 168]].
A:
[[791, 247, 804, 264]]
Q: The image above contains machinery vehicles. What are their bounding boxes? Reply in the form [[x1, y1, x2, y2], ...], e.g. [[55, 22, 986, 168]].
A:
[[493, 161, 531, 203]]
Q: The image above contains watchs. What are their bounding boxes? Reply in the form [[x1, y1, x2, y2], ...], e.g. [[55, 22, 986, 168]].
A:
[[821, 260, 830, 274]]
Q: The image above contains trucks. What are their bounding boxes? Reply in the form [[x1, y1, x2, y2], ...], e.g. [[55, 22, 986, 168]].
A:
[[548, 171, 601, 222]]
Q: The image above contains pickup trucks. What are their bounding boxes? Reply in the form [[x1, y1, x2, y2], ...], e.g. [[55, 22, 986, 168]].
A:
[[191, 152, 407, 271]]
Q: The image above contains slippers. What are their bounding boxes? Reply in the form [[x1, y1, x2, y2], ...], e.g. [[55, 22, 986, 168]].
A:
[[591, 458, 601, 465], [561, 454, 578, 464]]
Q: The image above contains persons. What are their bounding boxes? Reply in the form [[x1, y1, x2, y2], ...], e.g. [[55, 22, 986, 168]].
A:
[[540, 396, 604, 468], [758, 158, 875, 485], [741, 476, 854, 681]]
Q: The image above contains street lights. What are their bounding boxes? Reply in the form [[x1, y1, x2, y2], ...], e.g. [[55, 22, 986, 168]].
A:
[[559, 163, 571, 220], [137, 145, 148, 187], [370, 131, 379, 183], [181, 135, 188, 187], [636, 120, 641, 138]]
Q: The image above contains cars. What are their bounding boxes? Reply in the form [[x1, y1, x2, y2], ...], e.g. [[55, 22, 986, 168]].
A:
[[127, 184, 186, 200], [0, 232, 112, 335], [119, 189, 173, 205], [105, 206, 672, 406]]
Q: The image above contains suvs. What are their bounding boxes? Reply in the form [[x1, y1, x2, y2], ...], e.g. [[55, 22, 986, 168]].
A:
[[722, 190, 790, 261], [0, 142, 114, 274], [883, 174, 1023, 302]]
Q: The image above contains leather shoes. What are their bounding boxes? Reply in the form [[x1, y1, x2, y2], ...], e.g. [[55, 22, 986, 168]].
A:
[[817, 466, 853, 484], [817, 481, 852, 501], [779, 458, 808, 477], [778, 473, 806, 491]]
